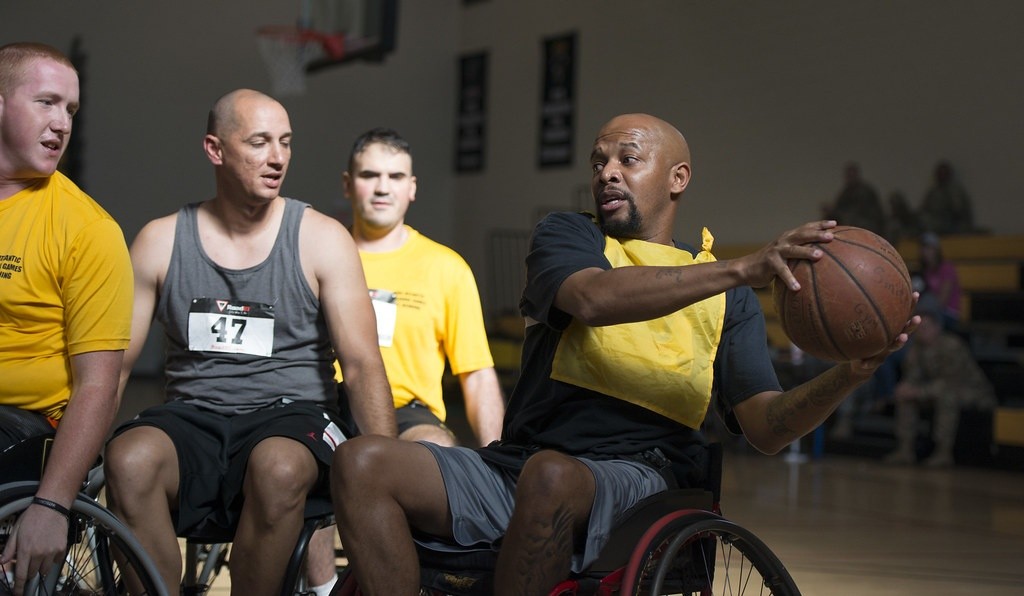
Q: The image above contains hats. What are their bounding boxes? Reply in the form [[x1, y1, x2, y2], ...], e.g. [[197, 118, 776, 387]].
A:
[[920, 228, 935, 253]]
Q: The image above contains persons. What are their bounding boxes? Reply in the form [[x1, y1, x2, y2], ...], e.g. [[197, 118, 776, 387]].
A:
[[779, 153, 993, 468], [107, 89, 397, 596], [332, 111, 920, 596], [0, 45, 134, 596], [305, 129, 504, 596]]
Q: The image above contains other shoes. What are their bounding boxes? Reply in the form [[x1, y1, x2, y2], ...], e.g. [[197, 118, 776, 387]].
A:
[[828, 420, 854, 442], [880, 446, 915, 466], [923, 454, 955, 471]]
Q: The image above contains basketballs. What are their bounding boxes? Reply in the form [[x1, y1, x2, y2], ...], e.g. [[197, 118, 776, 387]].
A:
[[779, 224, 914, 359]]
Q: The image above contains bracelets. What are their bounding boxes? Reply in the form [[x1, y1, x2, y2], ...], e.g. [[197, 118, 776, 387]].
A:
[[32, 497, 71, 518]]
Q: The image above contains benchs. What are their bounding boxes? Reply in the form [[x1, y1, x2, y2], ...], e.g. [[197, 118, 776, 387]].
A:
[[714, 232, 1024, 458], [441, 312, 525, 387]]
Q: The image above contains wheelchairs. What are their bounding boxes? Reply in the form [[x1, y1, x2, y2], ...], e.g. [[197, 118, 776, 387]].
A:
[[327, 503, 803, 596], [0, 431, 171, 596], [78, 464, 336, 596]]
